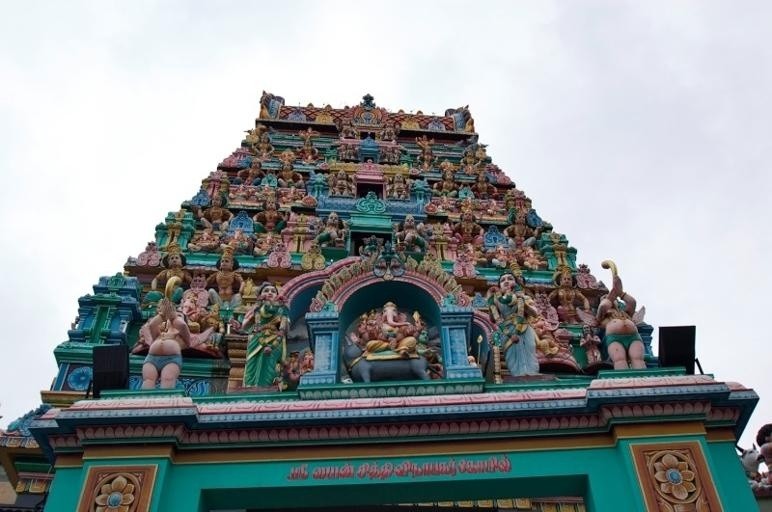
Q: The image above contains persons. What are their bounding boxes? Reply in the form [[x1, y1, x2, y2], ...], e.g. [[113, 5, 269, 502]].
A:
[[117, 95, 650, 393]]
